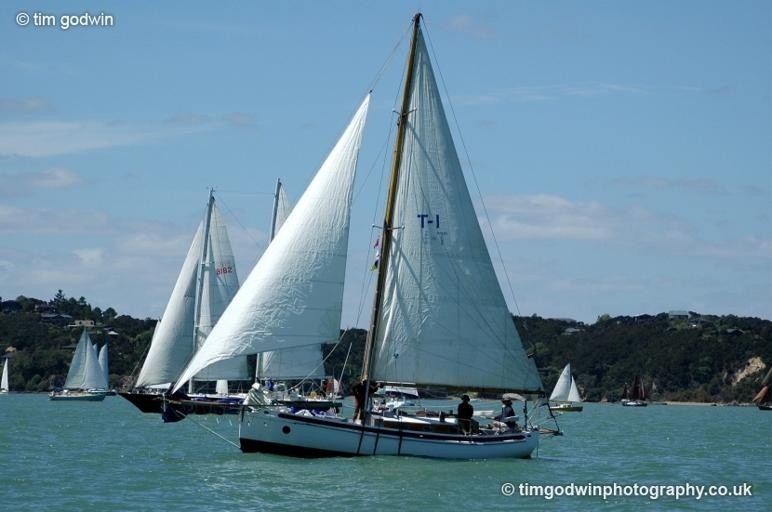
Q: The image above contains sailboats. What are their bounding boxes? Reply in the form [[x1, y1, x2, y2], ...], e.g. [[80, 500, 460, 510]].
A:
[[548, 363, 583, 412], [114, 174, 344, 416], [0, 357, 9, 395], [50, 328, 116, 401], [755, 367, 772, 410], [172, 11, 543, 460], [620, 374, 647, 406]]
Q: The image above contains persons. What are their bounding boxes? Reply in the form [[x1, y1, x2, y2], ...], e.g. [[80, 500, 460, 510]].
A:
[[256, 366, 516, 446], [48, 380, 62, 397]]
